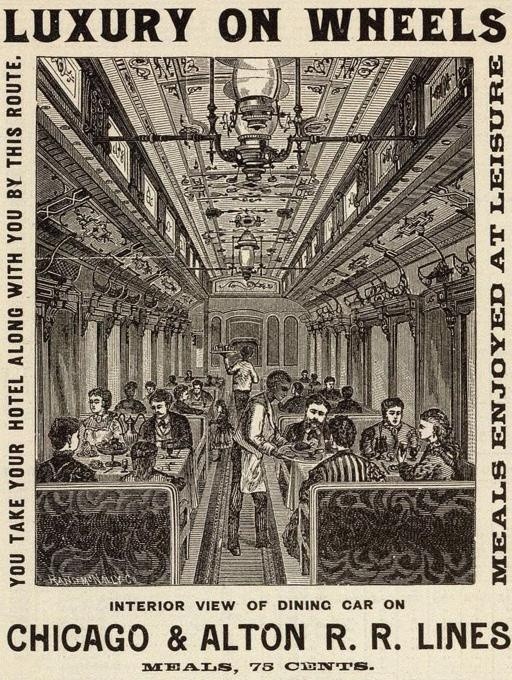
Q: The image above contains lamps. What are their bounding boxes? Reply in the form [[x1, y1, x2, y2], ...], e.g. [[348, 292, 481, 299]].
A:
[[228, 229, 267, 281], [202, 55, 306, 182]]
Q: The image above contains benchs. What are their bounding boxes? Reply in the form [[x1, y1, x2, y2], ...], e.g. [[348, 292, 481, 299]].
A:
[[34, 479, 189, 586], [80, 415, 208, 504], [280, 414, 387, 448], [310, 480, 473, 584]]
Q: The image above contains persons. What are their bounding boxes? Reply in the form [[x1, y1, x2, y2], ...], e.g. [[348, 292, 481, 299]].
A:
[[34, 345, 462, 576]]
